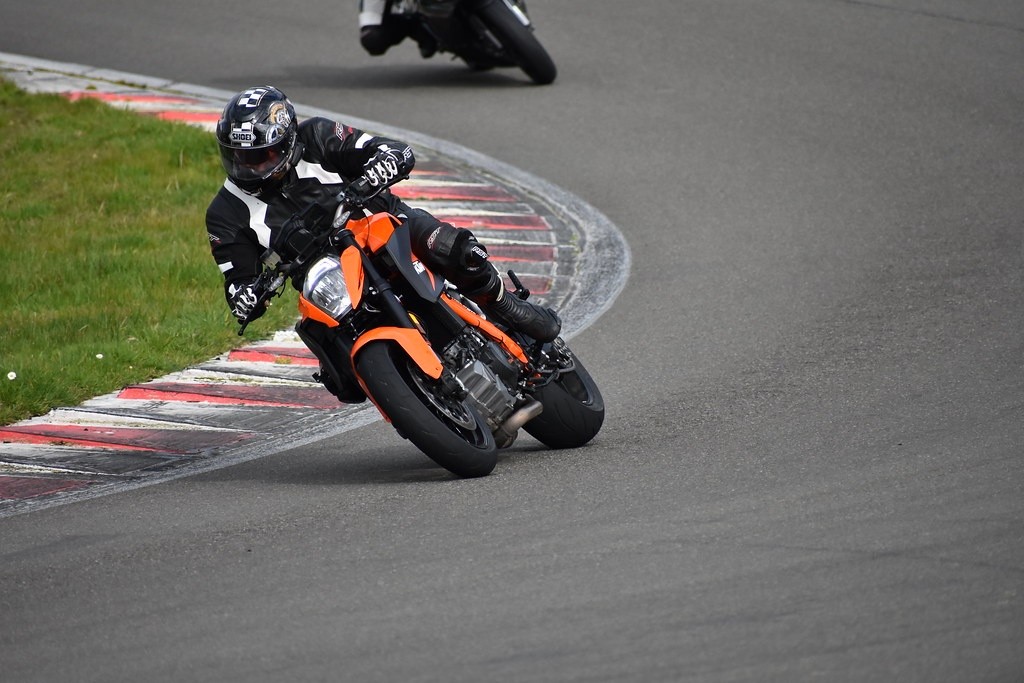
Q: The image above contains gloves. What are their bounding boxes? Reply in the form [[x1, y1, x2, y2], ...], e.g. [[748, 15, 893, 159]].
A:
[[362, 148, 406, 186], [226, 280, 270, 320]]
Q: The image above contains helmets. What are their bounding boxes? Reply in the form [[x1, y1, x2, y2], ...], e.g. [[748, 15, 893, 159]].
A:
[[216, 85, 299, 192]]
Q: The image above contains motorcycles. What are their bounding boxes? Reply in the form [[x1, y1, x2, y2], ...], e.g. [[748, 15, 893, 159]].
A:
[[226, 173, 607, 478], [360, 0, 559, 84]]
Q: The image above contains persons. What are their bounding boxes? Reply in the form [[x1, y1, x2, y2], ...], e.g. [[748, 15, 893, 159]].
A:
[[357, 1, 438, 59], [205, 86, 561, 403]]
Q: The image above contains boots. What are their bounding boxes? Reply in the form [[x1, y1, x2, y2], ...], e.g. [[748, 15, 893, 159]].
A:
[[468, 261, 562, 343]]
